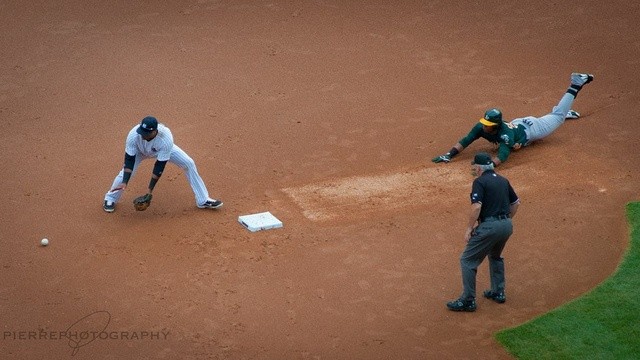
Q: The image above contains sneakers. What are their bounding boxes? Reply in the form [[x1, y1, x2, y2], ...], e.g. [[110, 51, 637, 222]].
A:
[[447, 298, 477, 312], [197, 197, 223, 208], [571, 72, 595, 86], [552, 106, 581, 119], [103, 200, 115, 212], [483, 289, 506, 304]]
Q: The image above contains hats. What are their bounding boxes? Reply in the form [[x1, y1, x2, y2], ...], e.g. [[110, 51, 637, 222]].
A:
[[480, 108, 503, 130], [470, 152, 492, 165], [136, 116, 158, 137]]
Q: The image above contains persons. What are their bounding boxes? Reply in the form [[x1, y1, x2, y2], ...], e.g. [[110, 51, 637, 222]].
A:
[[102, 116, 223, 212], [431, 73, 594, 174], [446, 153, 520, 312]]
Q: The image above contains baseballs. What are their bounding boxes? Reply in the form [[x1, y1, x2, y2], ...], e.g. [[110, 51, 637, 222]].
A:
[[41, 238, 49, 246]]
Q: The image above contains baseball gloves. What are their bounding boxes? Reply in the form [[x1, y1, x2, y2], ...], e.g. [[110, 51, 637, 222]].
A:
[[133, 193, 152, 211]]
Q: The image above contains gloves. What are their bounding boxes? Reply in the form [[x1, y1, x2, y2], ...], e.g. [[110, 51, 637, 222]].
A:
[[470, 163, 494, 176], [431, 152, 454, 164]]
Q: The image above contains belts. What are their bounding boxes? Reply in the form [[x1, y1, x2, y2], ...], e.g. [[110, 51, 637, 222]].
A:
[[481, 215, 510, 223]]
